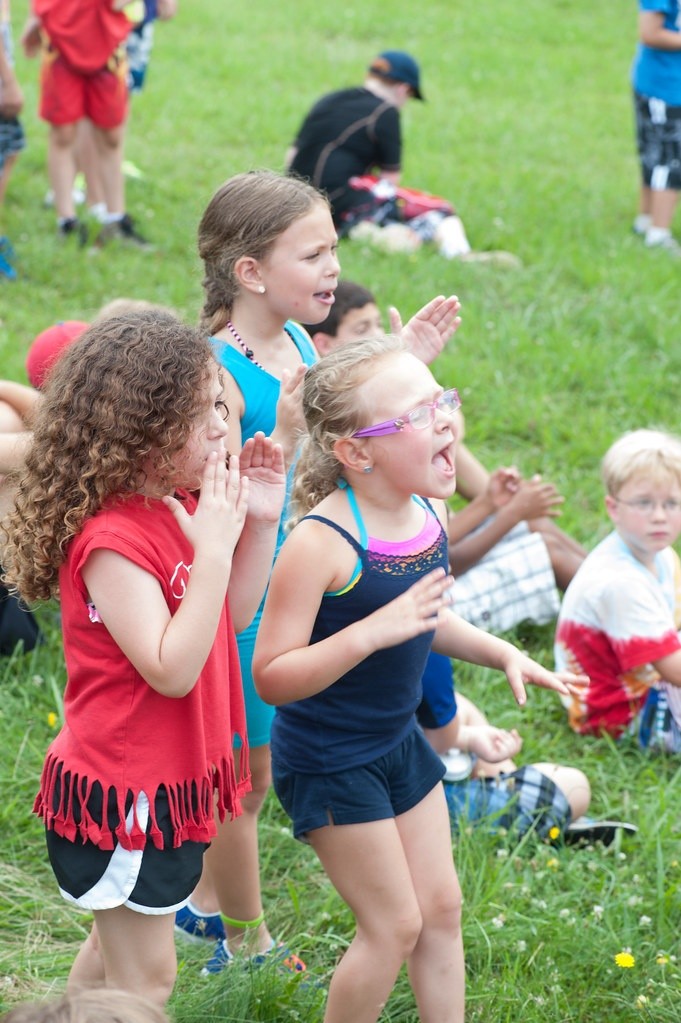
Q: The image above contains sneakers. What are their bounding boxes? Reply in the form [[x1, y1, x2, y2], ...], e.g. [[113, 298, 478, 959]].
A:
[[202, 939, 321, 988], [175, 902, 225, 942]]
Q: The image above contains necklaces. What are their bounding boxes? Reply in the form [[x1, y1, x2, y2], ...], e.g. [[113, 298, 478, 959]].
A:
[[227, 321, 267, 370]]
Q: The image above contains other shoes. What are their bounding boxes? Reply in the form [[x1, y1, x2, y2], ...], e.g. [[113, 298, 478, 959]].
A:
[[566, 823, 640, 847], [55, 219, 103, 254], [95, 212, 154, 250], [635, 218, 680, 250]]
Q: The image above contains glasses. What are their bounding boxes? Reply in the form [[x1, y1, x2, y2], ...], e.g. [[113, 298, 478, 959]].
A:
[[609, 492, 681, 515], [352, 386, 461, 439]]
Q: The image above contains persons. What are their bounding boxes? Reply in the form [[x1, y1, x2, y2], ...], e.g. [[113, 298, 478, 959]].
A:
[[0, 171, 638, 1023], [283, 50, 525, 272], [0, 0, 176, 279], [0, 990, 167, 1023], [553, 428, 681, 752], [629, 0, 681, 250]]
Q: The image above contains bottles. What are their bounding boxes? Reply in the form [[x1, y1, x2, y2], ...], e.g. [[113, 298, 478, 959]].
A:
[[442, 747, 472, 820]]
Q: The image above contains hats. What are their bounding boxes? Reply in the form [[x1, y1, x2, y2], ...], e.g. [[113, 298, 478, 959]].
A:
[[369, 51, 425, 103]]
